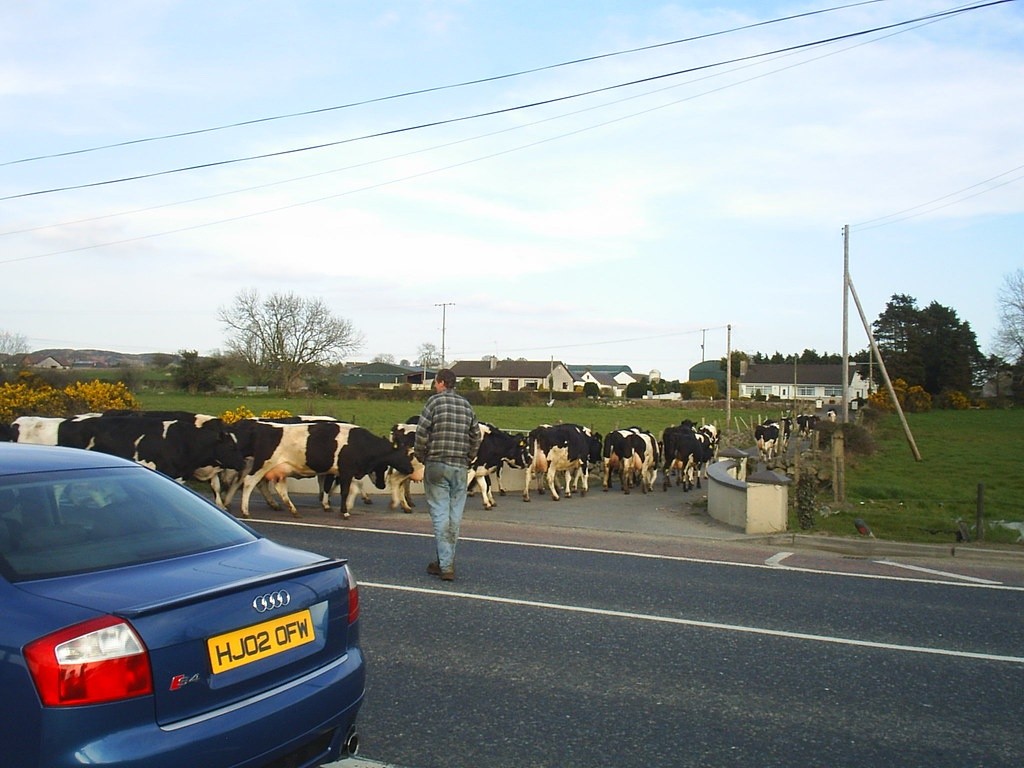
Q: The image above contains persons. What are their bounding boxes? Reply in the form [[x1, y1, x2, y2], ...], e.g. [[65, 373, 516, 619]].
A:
[[414, 368, 481, 580]]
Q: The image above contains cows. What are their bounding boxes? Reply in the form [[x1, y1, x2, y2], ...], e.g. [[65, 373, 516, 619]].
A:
[[0, 407, 838, 520]]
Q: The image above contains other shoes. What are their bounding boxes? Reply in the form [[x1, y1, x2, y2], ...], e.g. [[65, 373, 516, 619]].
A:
[[441, 572, 454, 580], [427, 562, 441, 574]]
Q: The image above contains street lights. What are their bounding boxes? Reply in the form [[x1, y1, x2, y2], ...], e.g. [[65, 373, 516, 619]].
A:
[[433, 302, 459, 372]]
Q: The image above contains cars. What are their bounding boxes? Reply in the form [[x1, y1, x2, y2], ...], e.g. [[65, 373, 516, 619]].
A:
[[1, 439, 369, 768]]
